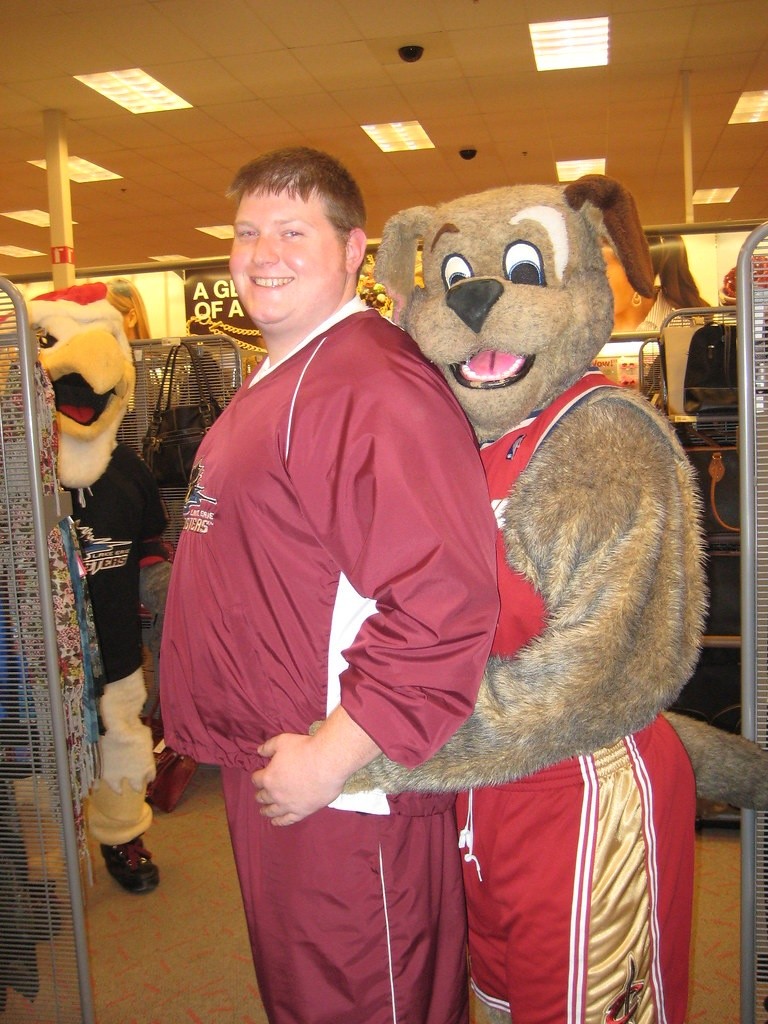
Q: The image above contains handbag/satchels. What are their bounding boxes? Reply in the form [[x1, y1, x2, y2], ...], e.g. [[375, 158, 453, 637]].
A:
[[684, 450, 745, 536], [682, 322, 740, 416], [140, 344, 223, 487], [147, 737, 198, 812]]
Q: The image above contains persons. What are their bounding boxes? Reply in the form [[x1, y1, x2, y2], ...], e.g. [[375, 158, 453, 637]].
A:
[[162, 148, 497, 1024], [107, 277, 162, 411], [601, 233, 712, 351]]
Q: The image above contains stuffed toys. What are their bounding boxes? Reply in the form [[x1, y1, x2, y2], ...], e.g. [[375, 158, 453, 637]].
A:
[[2, 285, 158, 894], [309, 174, 707, 1023]]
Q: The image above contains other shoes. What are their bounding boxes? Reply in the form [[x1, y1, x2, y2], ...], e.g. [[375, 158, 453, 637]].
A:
[[101, 841, 161, 892]]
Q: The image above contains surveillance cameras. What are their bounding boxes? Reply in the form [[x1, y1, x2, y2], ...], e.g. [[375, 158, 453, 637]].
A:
[[459, 150, 476, 160], [399, 46, 424, 63]]
